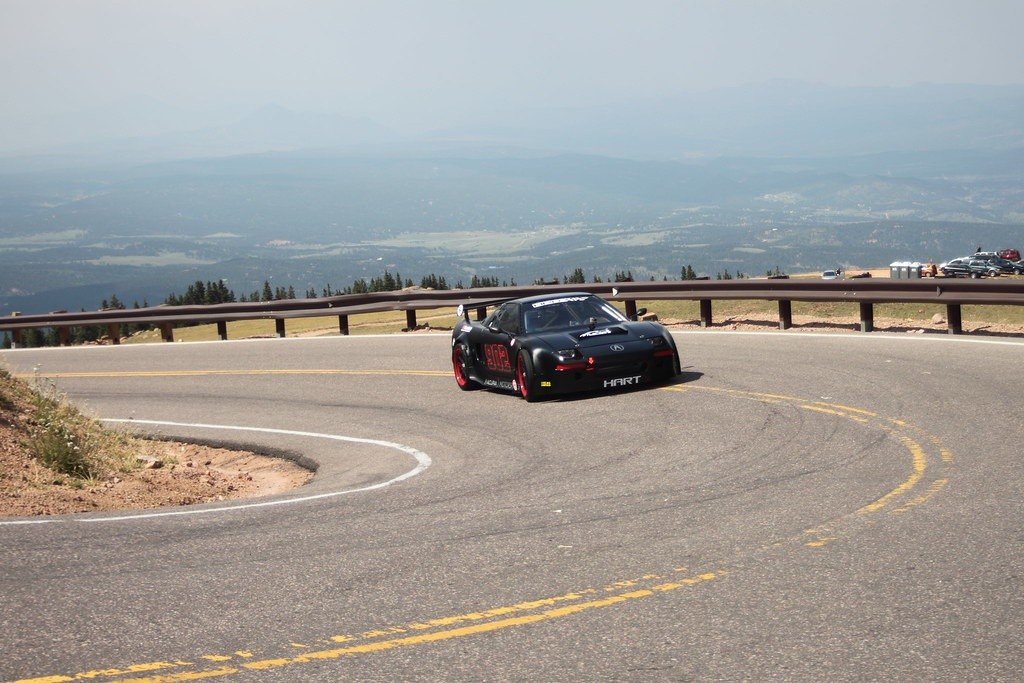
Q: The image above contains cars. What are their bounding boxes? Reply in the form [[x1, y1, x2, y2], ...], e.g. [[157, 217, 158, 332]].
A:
[[822, 269, 837, 280]]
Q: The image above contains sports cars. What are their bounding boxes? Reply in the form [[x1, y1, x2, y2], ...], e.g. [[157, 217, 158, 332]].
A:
[[450, 291, 685, 402]]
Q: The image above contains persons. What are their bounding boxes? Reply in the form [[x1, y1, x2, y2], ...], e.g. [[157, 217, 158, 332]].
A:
[[836, 268, 840, 275], [977, 245, 981, 252]]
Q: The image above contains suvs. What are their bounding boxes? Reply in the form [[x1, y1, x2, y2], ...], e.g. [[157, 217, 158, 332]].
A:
[[921, 248, 1024, 280]]
